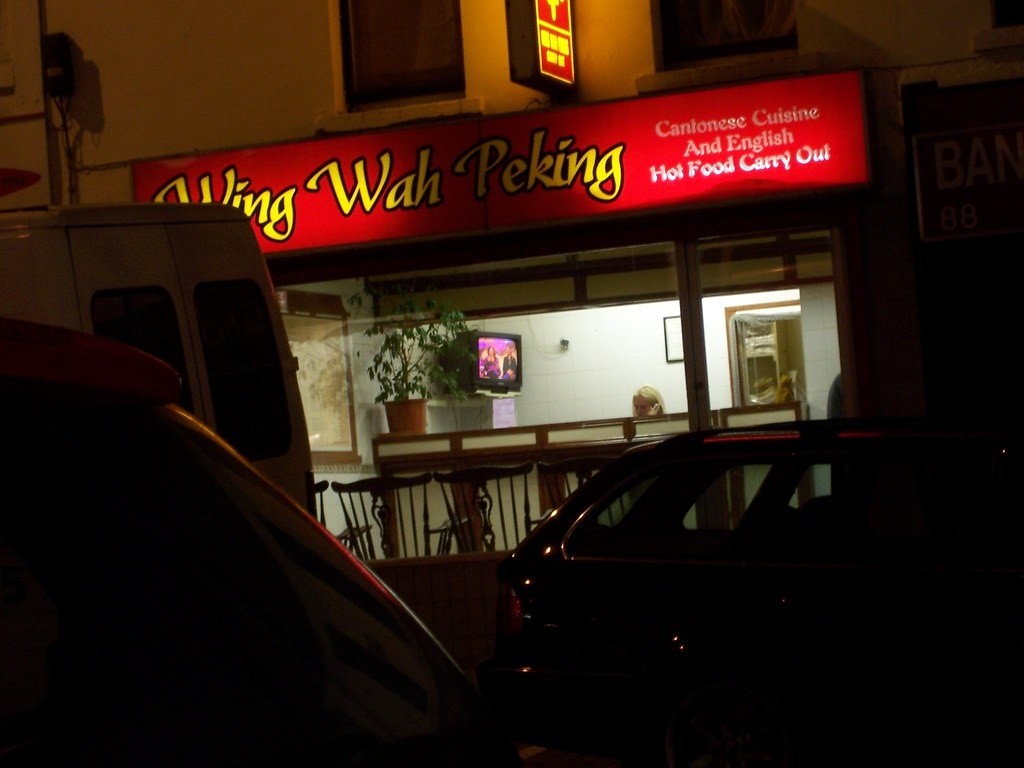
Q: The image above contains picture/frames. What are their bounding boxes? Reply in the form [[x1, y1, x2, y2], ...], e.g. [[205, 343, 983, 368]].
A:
[[663, 315, 686, 364]]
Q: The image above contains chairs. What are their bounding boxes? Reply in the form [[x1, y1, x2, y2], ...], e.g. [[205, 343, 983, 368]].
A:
[[536, 458, 638, 528], [331, 473, 434, 559], [432, 462, 534, 558]]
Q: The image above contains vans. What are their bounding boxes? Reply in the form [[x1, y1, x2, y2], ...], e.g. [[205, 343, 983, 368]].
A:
[[1, 203, 316, 520]]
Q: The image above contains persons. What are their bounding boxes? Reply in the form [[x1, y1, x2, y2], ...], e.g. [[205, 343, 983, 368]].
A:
[[478, 343, 517, 380], [826, 372, 844, 419], [632, 385, 665, 417]]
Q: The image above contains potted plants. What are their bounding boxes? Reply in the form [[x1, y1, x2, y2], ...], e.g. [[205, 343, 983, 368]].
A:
[[347, 288, 480, 435]]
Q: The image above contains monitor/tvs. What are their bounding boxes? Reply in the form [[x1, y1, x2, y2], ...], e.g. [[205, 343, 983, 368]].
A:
[[433, 330, 523, 393]]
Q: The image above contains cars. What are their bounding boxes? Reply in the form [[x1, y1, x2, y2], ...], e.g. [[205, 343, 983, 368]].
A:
[[1, 316, 522, 768], [499, 418, 1023, 768]]
